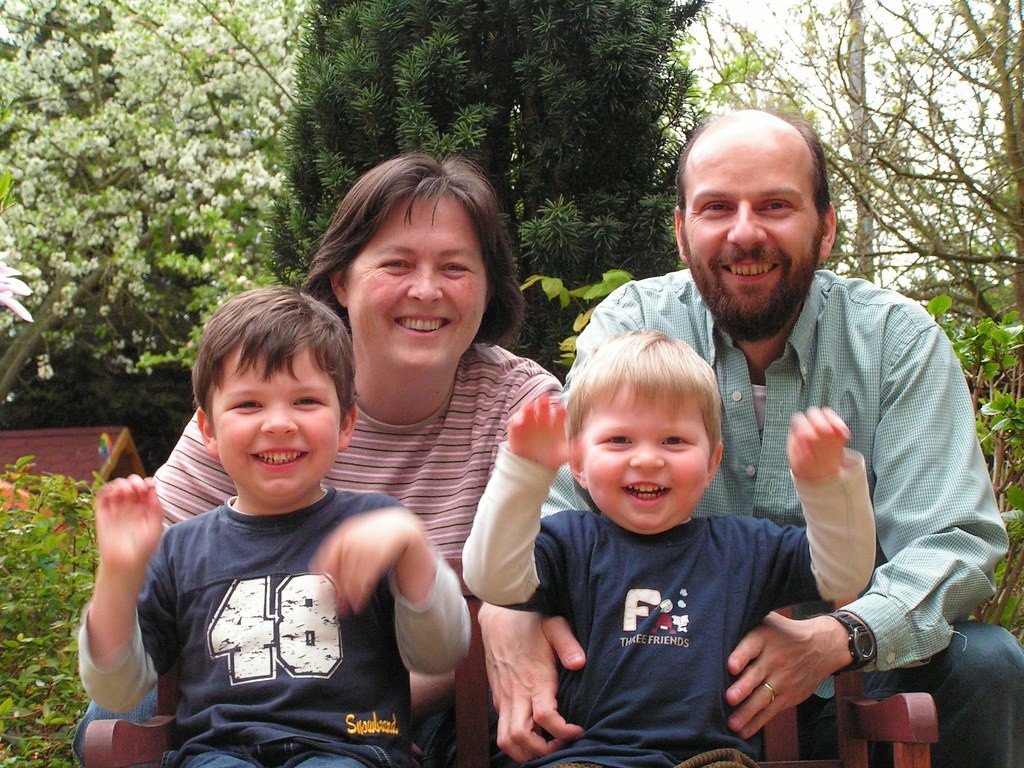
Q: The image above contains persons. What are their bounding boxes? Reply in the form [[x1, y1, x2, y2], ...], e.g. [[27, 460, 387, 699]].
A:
[[74, 152, 565, 768], [77, 285, 472, 768], [463, 330, 877, 768], [478, 110, 1024, 768]]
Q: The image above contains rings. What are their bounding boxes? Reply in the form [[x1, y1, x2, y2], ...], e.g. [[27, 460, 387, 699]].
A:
[[763, 683, 775, 703]]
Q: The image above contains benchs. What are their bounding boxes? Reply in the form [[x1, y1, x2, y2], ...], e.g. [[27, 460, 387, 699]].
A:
[[85, 561, 937, 768]]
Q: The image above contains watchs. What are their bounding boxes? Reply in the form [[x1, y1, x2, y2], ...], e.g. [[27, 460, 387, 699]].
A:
[[827, 613, 877, 676]]
